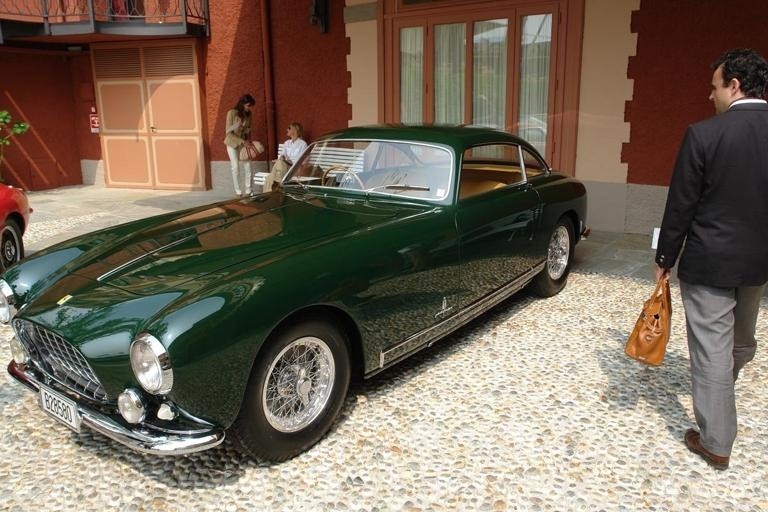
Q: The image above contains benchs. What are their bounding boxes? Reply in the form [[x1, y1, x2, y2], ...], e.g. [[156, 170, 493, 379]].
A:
[[253, 143, 366, 186]]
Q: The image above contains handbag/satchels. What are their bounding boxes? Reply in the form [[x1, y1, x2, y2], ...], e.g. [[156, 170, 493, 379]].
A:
[[239, 140, 265, 161], [271, 155, 293, 183], [625, 268, 672, 366]]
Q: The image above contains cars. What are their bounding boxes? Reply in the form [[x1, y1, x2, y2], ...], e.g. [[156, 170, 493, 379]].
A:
[[473, 111, 546, 162], [0, 123, 590, 462], [0, 182, 33, 275]]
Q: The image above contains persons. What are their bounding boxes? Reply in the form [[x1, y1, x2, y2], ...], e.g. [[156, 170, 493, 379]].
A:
[[654, 46, 766, 469], [264, 123, 306, 193], [223, 94, 258, 200]]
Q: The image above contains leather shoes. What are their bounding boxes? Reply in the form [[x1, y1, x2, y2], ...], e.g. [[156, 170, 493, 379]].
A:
[[685, 431, 729, 470]]
